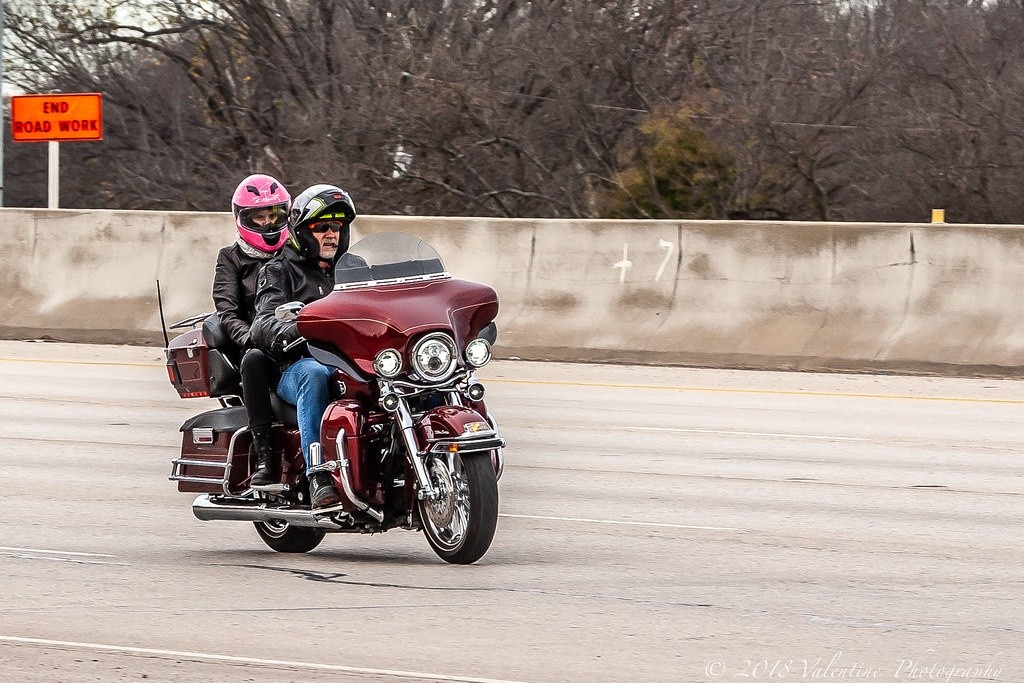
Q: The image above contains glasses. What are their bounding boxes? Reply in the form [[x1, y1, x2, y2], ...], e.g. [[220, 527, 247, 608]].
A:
[[310, 223, 341, 234]]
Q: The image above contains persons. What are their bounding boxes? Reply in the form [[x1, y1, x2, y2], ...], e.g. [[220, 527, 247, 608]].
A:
[[250, 184, 355, 510], [213, 173, 300, 485]]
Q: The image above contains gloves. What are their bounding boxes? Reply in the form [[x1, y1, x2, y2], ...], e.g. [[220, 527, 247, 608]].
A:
[[276, 321, 300, 344]]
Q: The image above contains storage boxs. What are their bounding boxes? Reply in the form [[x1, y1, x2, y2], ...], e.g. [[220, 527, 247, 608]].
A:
[[166, 330, 211, 398], [177, 405, 253, 492]]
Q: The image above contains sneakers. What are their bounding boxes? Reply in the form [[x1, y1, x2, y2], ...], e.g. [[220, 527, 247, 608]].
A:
[[250, 462, 283, 485], [308, 471, 340, 509]]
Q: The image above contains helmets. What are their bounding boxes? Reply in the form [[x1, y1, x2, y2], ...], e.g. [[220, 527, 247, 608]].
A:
[[231, 173, 290, 251], [288, 184, 356, 259]]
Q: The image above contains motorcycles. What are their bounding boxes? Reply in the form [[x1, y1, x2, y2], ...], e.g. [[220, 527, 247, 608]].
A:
[[164, 233, 508, 565]]
